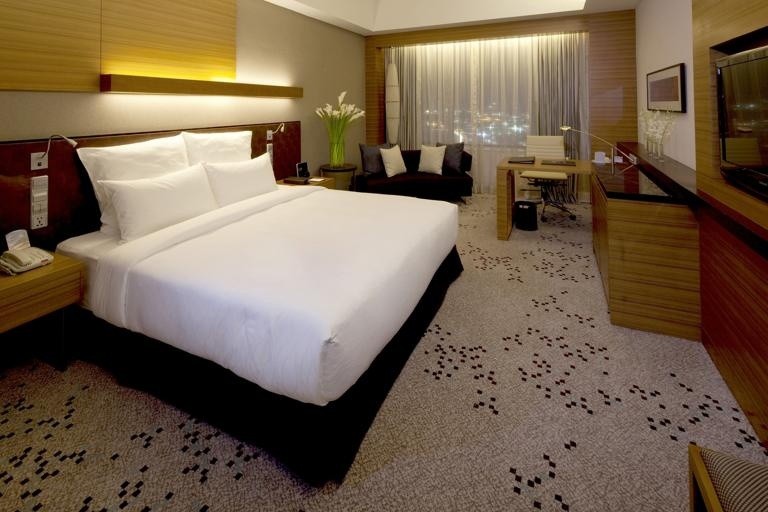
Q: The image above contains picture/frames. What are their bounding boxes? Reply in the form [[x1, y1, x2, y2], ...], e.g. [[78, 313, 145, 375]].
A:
[[645, 63, 686, 113]]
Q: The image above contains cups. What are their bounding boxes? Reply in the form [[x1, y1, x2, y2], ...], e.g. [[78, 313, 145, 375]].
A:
[[594, 151, 606, 162]]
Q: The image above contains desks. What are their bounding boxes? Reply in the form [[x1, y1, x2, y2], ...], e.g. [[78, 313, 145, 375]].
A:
[[495, 155, 592, 241]]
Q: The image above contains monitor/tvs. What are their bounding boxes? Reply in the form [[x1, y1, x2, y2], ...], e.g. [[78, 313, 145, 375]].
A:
[[714, 46, 768, 203]]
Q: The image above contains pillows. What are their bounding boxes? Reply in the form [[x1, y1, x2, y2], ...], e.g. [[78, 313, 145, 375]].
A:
[[96, 163, 219, 247], [202, 152, 280, 207], [436, 141, 465, 176], [417, 143, 447, 177], [75, 133, 190, 239], [378, 144, 409, 179], [179, 130, 255, 166], [357, 141, 391, 179]]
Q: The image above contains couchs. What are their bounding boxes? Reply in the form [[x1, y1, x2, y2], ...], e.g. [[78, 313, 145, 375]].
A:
[[349, 149, 474, 205]]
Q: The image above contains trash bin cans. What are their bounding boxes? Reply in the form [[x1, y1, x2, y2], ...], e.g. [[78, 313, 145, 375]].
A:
[[514, 200, 537, 231]]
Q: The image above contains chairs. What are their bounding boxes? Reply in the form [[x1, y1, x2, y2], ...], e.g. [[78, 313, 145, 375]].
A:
[[519, 135, 577, 224]]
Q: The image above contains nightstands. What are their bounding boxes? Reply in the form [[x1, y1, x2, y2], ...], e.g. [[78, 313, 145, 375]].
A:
[[275, 175, 337, 194], [0, 251, 87, 370]]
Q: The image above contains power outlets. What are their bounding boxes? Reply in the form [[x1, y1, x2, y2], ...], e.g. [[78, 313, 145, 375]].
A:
[[32, 212, 49, 229]]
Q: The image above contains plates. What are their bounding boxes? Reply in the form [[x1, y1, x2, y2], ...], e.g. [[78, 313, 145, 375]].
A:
[[591, 160, 612, 165]]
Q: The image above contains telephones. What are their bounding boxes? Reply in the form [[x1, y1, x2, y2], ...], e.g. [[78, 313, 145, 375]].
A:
[[0, 247, 54, 276]]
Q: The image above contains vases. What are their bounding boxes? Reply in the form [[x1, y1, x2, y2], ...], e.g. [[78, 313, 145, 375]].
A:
[[328, 140, 346, 167]]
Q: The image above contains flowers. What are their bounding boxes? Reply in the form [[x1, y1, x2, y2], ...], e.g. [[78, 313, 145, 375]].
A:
[[314, 90, 367, 142]]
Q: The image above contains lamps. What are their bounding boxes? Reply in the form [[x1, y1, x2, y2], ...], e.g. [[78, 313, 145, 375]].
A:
[[559, 125, 634, 175]]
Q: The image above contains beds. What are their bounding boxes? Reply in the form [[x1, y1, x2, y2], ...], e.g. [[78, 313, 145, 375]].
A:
[[55, 184, 465, 490]]
